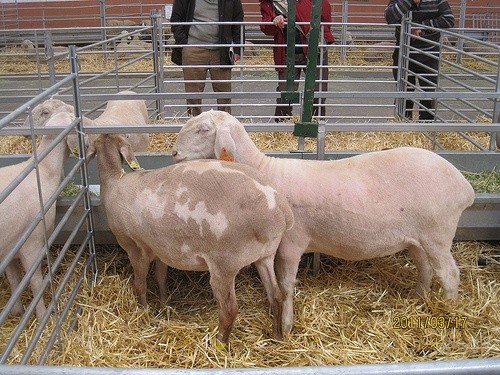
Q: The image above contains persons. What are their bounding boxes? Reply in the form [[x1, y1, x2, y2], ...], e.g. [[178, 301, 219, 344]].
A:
[[384, 0, 455, 123], [259, 0, 335, 123], [171, 0, 245, 118]]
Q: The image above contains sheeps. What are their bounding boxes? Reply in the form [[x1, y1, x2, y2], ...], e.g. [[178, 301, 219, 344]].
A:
[[362, 41, 396, 61], [172, 108, 476, 315], [20, 39, 81, 71], [23, 90, 150, 158], [0, 112, 77, 327], [86, 132, 294, 352], [341, 30, 353, 51], [106, 18, 260, 60]]
